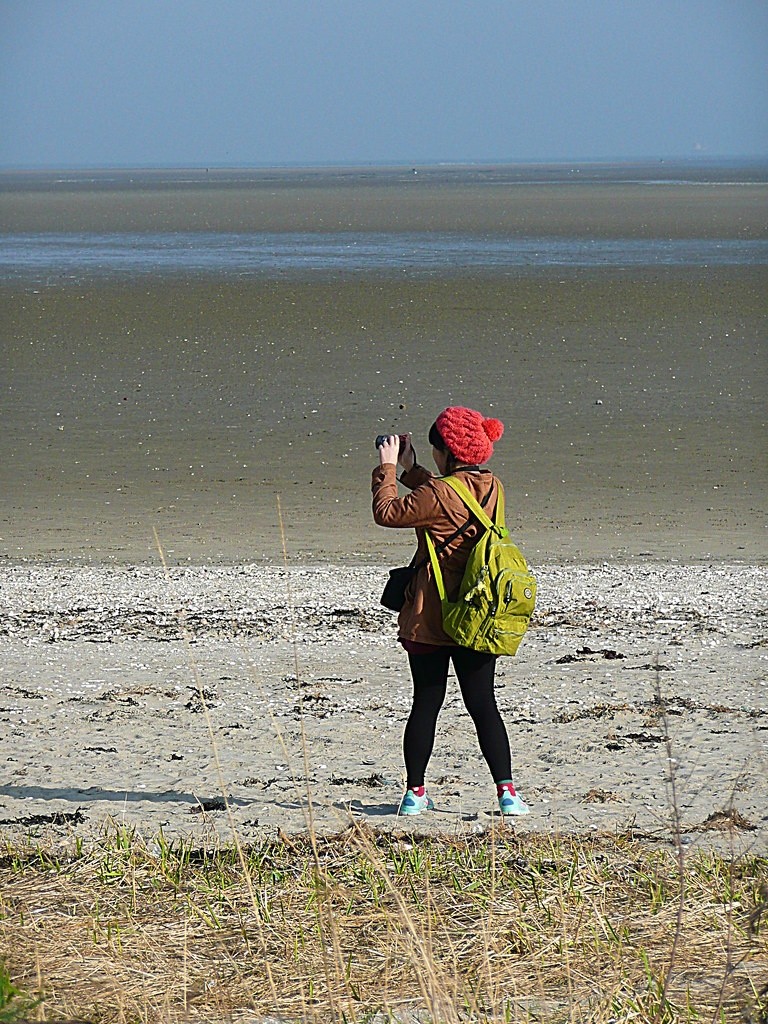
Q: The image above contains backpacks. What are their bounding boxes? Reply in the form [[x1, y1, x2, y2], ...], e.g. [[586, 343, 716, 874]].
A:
[[418, 472, 540, 659]]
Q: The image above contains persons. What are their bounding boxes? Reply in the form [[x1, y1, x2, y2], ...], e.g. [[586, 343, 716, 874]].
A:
[[370, 406, 531, 816]]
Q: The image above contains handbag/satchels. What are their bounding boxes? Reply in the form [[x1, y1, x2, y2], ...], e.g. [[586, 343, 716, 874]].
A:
[[377, 561, 415, 612]]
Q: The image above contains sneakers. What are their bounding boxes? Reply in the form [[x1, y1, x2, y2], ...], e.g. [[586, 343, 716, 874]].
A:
[[398, 788, 435, 818], [496, 785, 532, 817]]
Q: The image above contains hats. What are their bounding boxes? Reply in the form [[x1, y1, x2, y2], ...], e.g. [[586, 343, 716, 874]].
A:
[[435, 404, 506, 465]]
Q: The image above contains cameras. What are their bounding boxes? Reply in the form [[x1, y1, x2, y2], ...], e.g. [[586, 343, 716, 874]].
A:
[[375, 436, 406, 455]]
